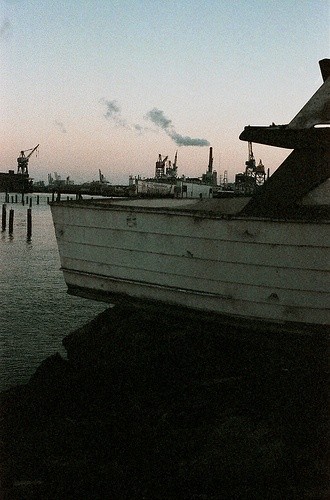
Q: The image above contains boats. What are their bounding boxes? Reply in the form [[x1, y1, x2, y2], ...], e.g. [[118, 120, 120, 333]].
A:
[[0, 144, 41, 193], [129, 153, 185, 199], [212, 140, 271, 198], [175, 146, 226, 200], [48, 60, 330, 340]]
